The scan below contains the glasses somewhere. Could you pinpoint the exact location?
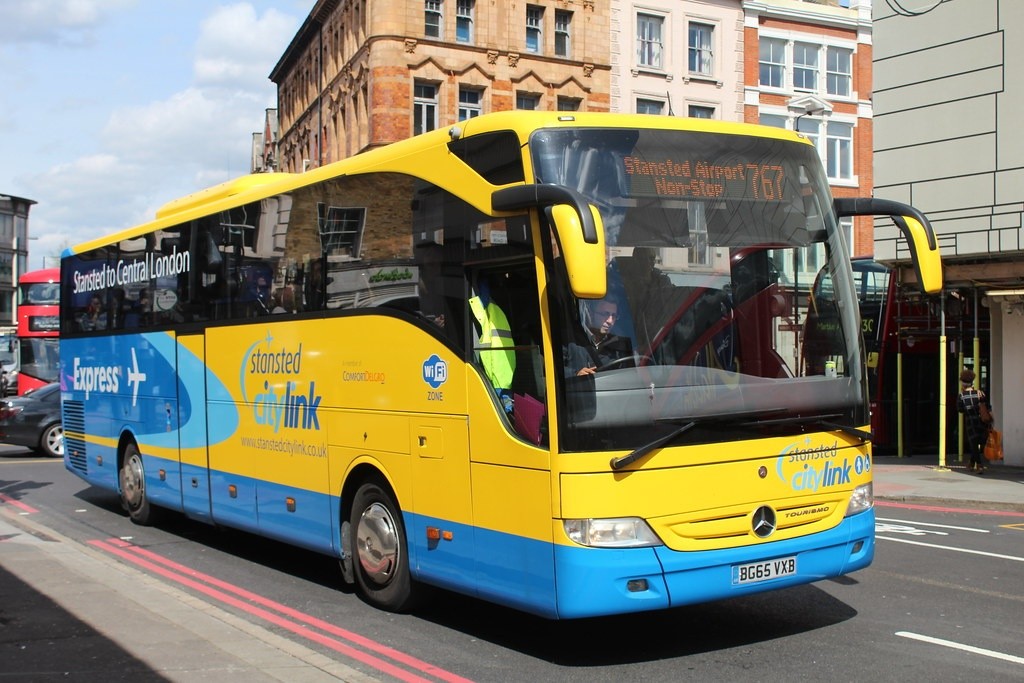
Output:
[593,311,621,321]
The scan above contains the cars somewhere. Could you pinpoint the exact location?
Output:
[0,382,64,458]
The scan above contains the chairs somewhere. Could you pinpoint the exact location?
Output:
[612,254,636,282]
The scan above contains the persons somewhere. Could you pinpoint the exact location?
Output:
[958,370,995,473]
[76,240,673,378]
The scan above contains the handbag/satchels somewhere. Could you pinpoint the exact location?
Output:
[984,427,1004,460]
[976,389,993,423]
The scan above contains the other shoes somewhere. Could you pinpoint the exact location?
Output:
[976,464,984,473]
[966,460,974,472]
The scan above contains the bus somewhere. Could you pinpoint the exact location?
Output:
[806,255,989,455]
[59,112,944,624]
[8,269,60,398]
